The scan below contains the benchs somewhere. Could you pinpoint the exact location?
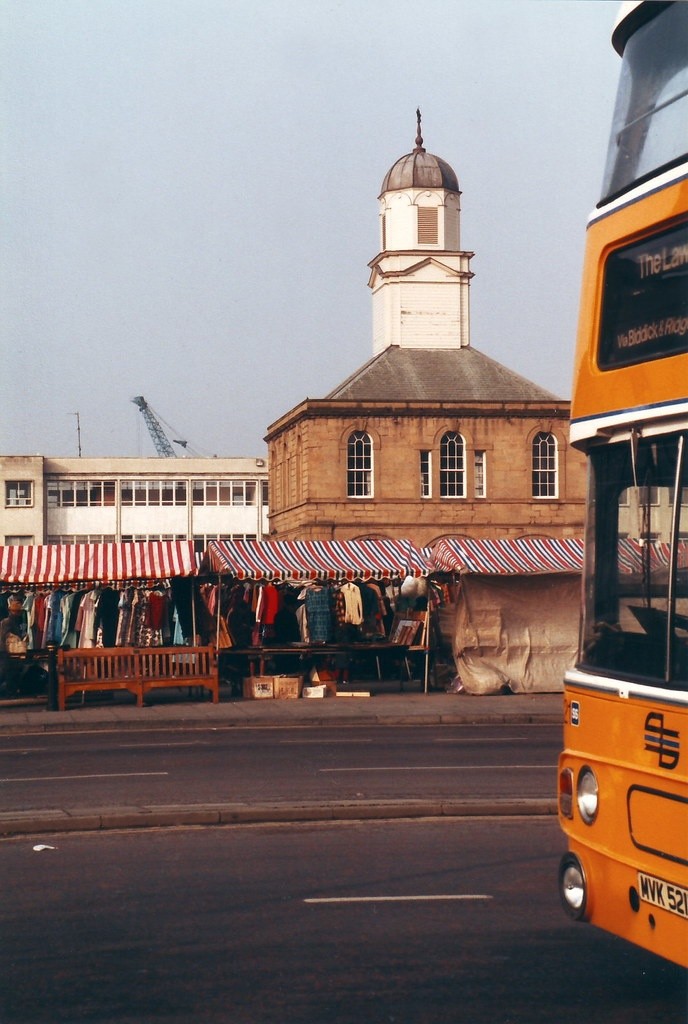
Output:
[58,644,219,710]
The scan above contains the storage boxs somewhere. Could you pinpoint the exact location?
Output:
[241,675,372,698]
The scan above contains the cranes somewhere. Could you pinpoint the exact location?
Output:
[132,393,204,454]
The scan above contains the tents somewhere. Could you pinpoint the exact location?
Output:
[432,538,688,696]
[0,540,197,666]
[203,538,431,694]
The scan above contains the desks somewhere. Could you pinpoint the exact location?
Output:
[222,643,424,691]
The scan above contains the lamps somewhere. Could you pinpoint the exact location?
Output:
[256,459,264,466]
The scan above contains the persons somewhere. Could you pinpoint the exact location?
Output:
[0,595,27,655]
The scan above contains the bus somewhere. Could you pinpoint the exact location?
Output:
[555,0,688,973]
